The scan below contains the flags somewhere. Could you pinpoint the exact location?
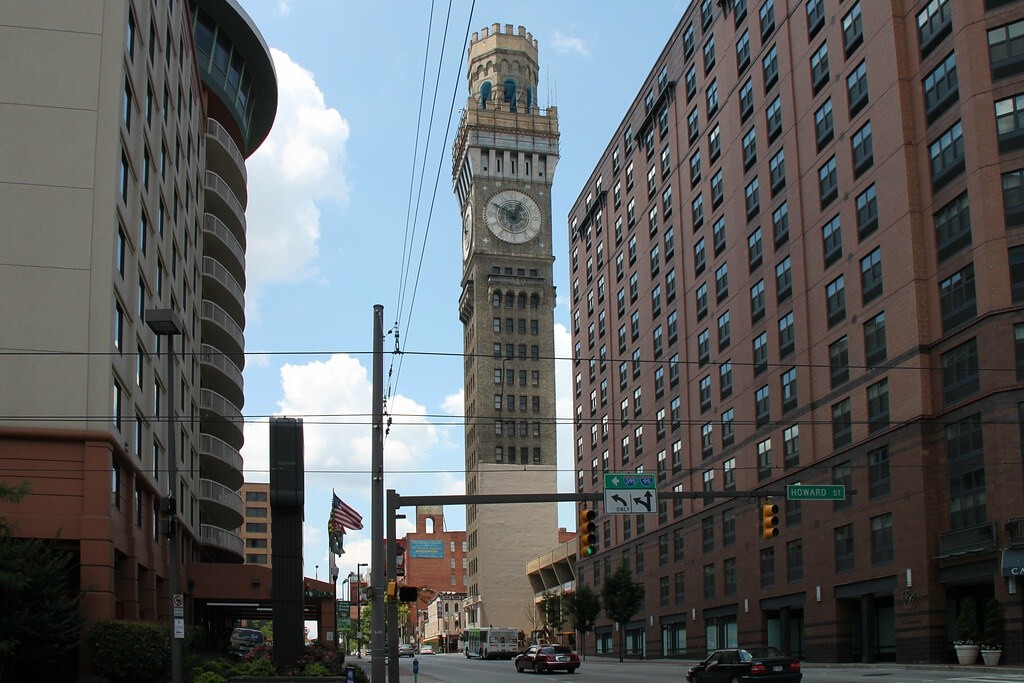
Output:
[333,492,364,530]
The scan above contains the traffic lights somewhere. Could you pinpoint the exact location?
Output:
[400,587,418,601]
[581,509,597,558]
[762,503,780,540]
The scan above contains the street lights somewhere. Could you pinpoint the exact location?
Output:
[358,563,367,658]
[142,309,183,683]
[422,588,446,654]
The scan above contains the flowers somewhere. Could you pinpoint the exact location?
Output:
[952,625,981,645]
[977,639,1002,650]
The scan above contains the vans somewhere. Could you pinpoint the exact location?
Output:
[229,629,266,658]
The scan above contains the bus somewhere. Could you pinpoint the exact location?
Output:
[461,624,518,661]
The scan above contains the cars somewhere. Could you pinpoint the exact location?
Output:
[421,645,433,654]
[515,644,581,673]
[686,647,803,683]
[399,643,415,658]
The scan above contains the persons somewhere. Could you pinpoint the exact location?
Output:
[518,629,526,646]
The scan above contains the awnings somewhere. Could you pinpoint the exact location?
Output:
[1001,548,1024,577]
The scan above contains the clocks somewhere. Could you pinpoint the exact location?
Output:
[462,200,473,263]
[482,188,546,246]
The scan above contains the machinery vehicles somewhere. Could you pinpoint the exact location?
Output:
[518,629,550,650]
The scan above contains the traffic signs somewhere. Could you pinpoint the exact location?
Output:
[787,484,846,499]
[604,474,659,514]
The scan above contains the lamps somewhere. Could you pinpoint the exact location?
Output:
[745,598,749,613]
[650,615,654,627]
[816,586,822,603]
[616,622,619,632]
[691,608,696,621]
[906,568,912,587]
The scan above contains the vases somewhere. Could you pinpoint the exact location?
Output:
[981,650,1003,667]
[954,644,981,665]
